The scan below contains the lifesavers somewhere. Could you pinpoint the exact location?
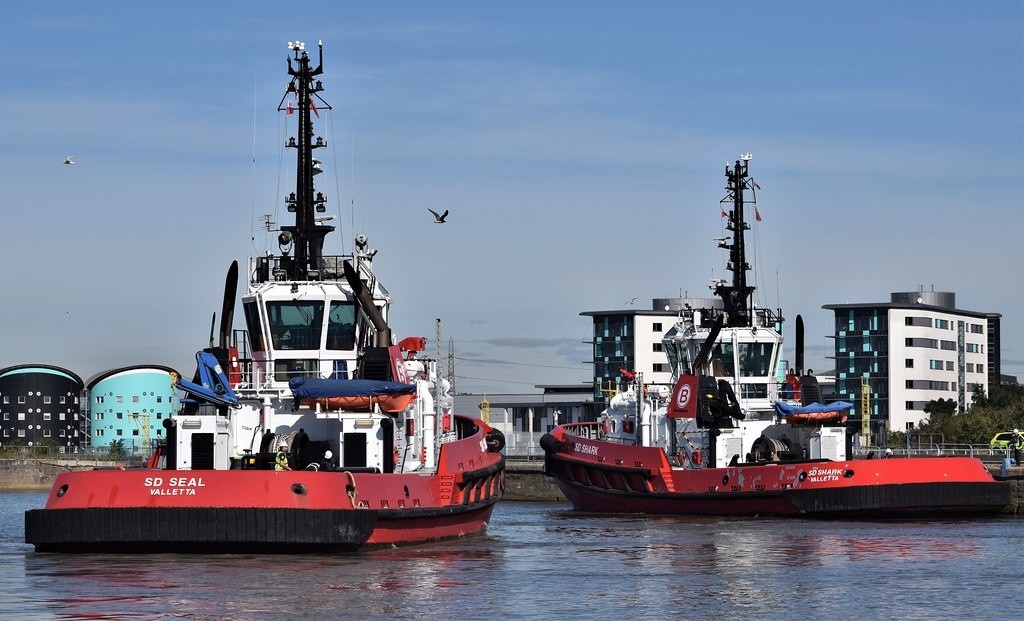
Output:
[600,420,608,434]
[675,451,684,464]
[421,362,427,380]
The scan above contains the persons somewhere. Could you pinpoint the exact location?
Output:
[1008,429,1024,467]
[275,441,292,470]
[867,451,874,459]
[321,451,335,472]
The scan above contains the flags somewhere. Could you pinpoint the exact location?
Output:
[756,211,762,221]
[753,181,761,190]
[288,101,293,114]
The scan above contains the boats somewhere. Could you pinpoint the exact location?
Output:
[781,407,851,427]
[300,385,419,416]
[26,36,509,560]
[540,154,1016,524]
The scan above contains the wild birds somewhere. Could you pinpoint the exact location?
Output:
[63,156,78,164]
[428,208,449,224]
[625,298,638,304]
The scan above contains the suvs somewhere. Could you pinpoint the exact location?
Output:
[988,430,1024,456]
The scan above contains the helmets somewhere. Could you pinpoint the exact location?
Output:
[279,441,288,447]
[1013,429,1018,433]
[886,448,891,453]
